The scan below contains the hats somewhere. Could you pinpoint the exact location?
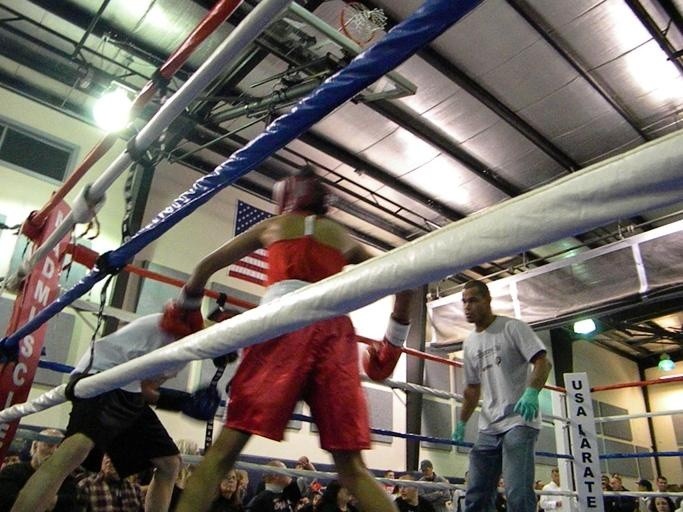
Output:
[420,461,431,470]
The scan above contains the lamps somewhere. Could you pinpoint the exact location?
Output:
[659,341,674,372]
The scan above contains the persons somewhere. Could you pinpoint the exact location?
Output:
[1,428,92,512]
[535,469,578,512]
[449,280,553,512]
[601,472,683,512]
[384,459,508,512]
[160,162,401,511]
[11,313,238,512]
[211,455,364,512]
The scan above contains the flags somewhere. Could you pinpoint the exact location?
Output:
[227,199,276,286]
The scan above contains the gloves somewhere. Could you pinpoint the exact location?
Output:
[514,386,541,421]
[159,285,205,338]
[155,386,217,417]
[363,315,413,380]
[452,421,464,442]
